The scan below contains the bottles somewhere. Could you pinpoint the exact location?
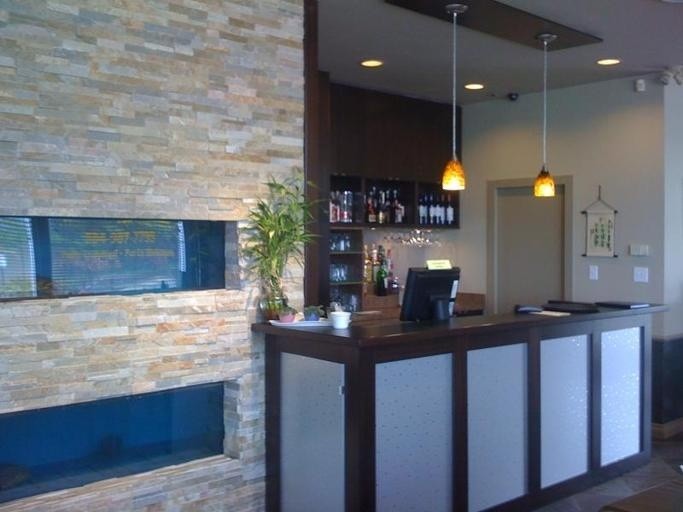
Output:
[439,195,444,225]
[330,264,349,282]
[330,234,351,251]
[370,250,381,283]
[418,194,424,227]
[329,191,353,223]
[378,191,390,225]
[392,188,404,225]
[446,195,455,226]
[434,195,441,225]
[364,245,372,283]
[429,196,435,225]
[376,259,388,296]
[424,193,429,225]
[367,197,377,225]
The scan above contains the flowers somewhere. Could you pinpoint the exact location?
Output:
[305,304,325,317]
[238,166,329,287]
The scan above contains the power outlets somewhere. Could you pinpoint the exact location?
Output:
[633,266,648,284]
[588,265,600,282]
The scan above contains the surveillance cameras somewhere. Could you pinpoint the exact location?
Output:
[508,92,518,100]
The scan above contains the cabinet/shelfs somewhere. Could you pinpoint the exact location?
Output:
[319,174,461,313]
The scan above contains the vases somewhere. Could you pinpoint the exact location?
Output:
[258,286,290,321]
[305,309,320,321]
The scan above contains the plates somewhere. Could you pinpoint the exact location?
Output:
[268,318,332,326]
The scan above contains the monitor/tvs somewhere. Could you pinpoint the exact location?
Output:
[399,267,460,323]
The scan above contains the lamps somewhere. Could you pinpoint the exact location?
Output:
[534,33,558,196]
[442,4,468,191]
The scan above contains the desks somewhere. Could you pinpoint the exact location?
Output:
[251,304,670,512]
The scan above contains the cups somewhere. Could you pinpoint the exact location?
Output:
[330,311,352,330]
[338,264,345,281]
[335,239,345,251]
[398,285,406,306]
[330,263,340,281]
